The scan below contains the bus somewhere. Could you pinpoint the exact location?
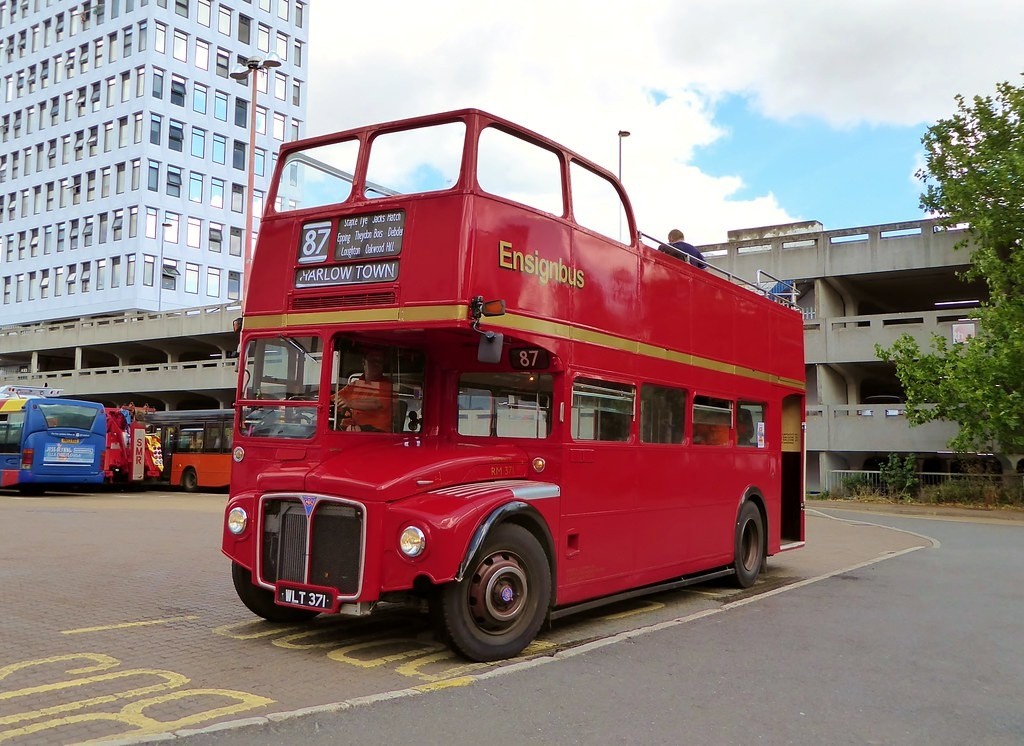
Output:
[0,385,107,497]
[142,410,312,493]
[219,107,809,665]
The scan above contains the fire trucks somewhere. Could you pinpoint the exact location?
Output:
[41,404,163,493]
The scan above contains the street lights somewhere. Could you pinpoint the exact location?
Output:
[228,50,281,429]
[158,222,173,319]
[617,131,631,184]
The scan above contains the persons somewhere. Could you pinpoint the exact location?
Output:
[330,351,396,433]
[658,229,709,271]
[693,402,754,446]
